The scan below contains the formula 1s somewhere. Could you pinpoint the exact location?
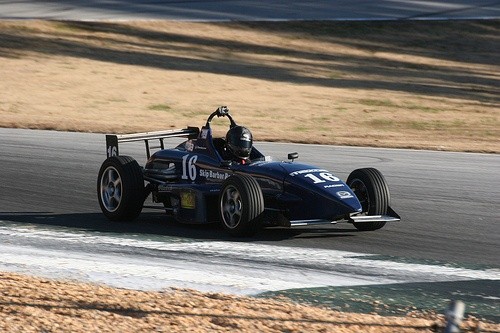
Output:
[96,105,403,237]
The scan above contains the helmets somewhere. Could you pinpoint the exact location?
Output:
[225,125,253,159]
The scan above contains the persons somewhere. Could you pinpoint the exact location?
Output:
[223,124,254,165]
